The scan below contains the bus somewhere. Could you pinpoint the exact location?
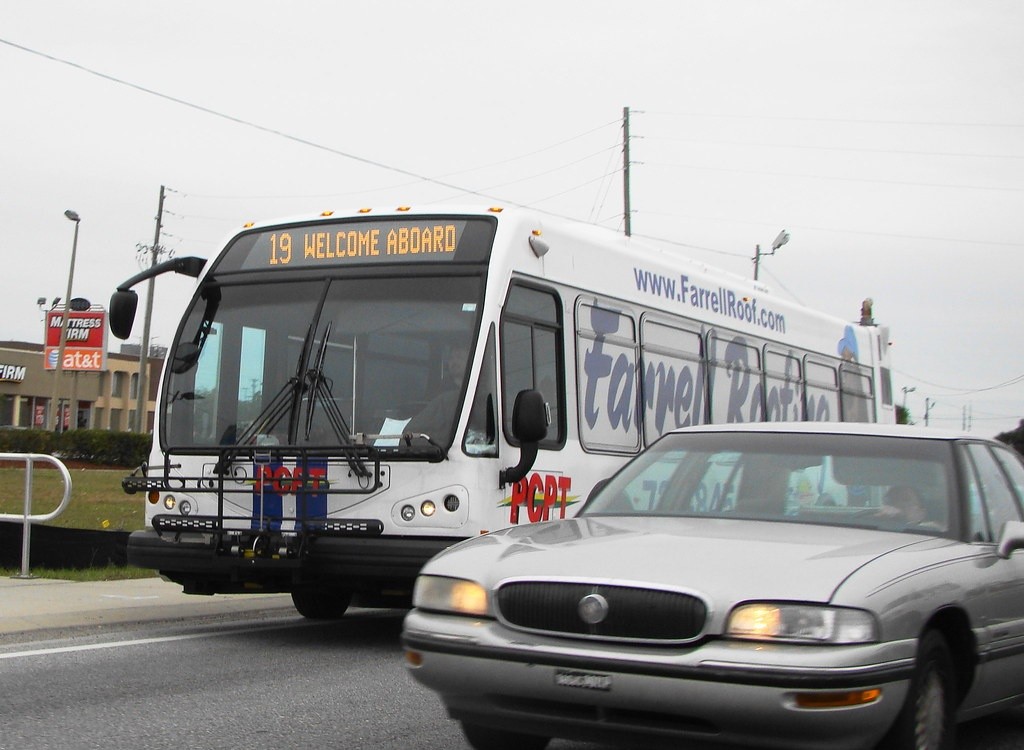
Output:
[110,207,891,630]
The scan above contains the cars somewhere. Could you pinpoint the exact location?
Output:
[401,421,1024,750]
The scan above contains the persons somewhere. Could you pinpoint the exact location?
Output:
[430,349,485,401]
[876,486,945,531]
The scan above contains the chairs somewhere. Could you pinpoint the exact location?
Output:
[738,467,789,517]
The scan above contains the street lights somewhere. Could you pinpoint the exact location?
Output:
[903,384,916,410]
[45,209,83,433]
[753,227,792,284]
[37,296,62,350]
